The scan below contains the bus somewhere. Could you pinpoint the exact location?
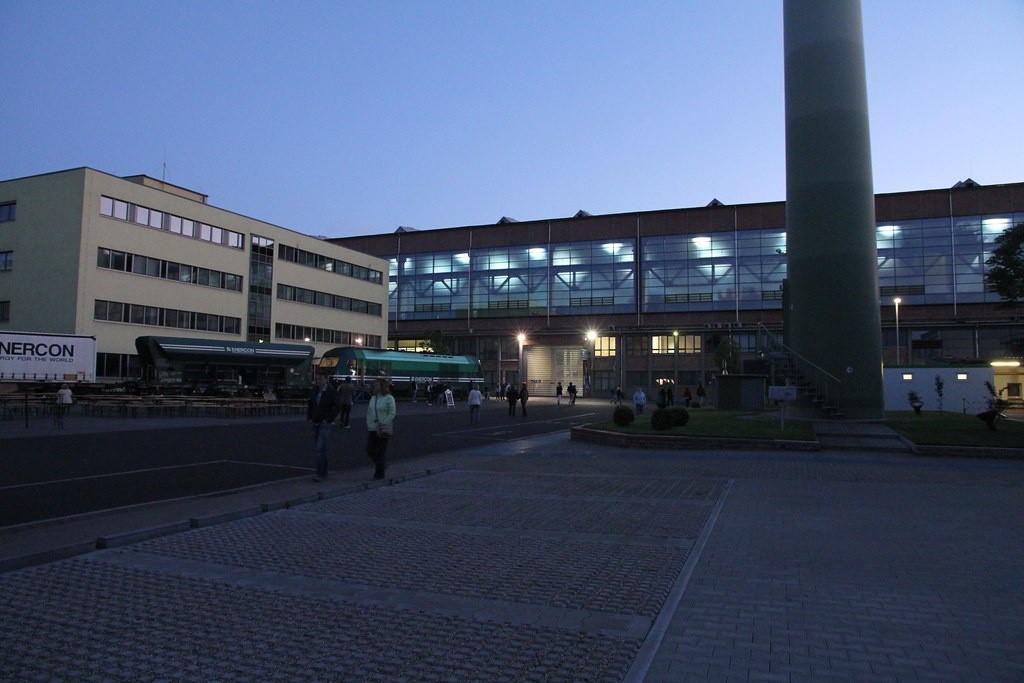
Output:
[131,335,485,400]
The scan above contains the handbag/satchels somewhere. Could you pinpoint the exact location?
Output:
[377,422,394,439]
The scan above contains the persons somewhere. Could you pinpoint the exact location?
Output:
[697,386,706,408]
[684,388,692,407]
[610,385,674,414]
[56,384,72,415]
[310,371,356,483]
[556,382,578,408]
[412,379,451,407]
[365,378,396,481]
[468,383,482,425]
[496,382,529,418]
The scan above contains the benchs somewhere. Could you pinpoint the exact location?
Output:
[0,394,308,420]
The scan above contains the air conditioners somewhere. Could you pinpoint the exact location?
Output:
[704,321,742,328]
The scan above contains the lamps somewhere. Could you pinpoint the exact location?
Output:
[776,249,783,254]
[911,401,923,414]
[977,410,998,431]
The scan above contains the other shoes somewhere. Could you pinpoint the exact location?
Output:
[343,425,352,429]
[312,475,321,481]
[371,473,385,481]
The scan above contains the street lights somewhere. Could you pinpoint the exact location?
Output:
[673,330,679,400]
[893,297,902,369]
